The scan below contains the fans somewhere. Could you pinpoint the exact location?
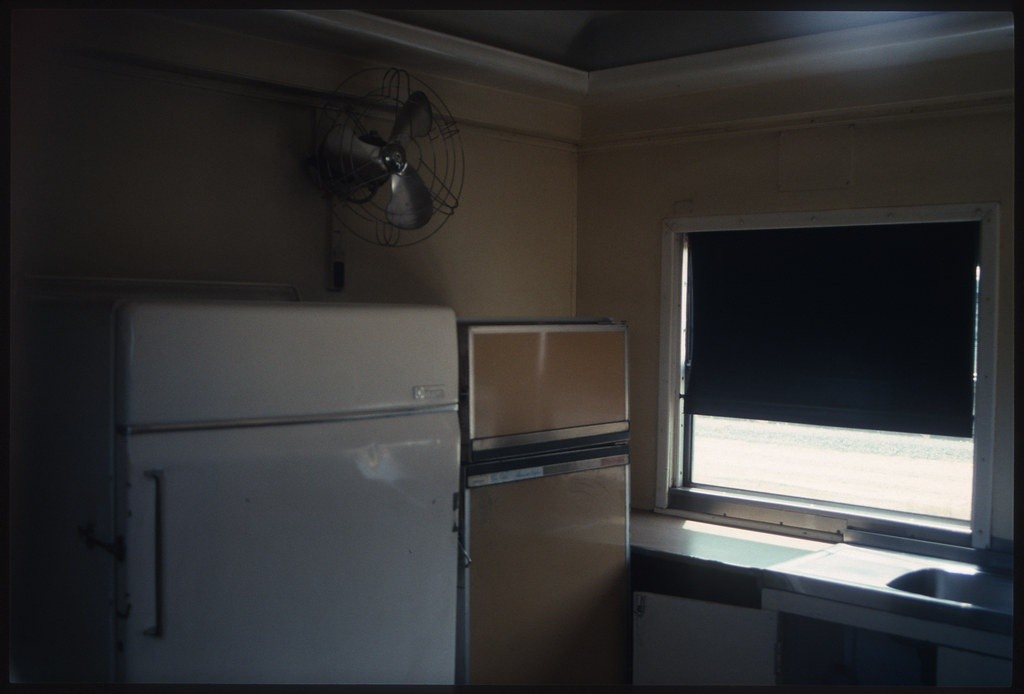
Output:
[311,64,466,290]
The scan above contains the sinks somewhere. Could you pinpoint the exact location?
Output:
[889,568,1013,611]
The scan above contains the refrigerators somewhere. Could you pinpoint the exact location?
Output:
[75,293,459,694]
